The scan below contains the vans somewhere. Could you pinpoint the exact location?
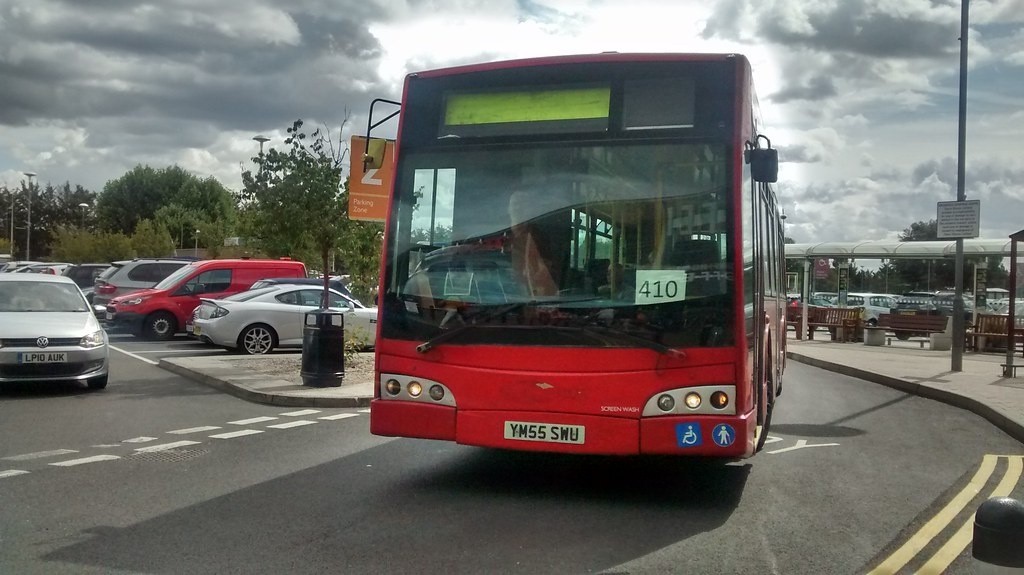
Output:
[105,256,307,342]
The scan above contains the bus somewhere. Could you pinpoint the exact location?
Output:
[343,49,798,476]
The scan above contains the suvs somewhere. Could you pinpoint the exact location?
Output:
[94,257,200,313]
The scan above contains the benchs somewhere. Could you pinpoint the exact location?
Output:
[807,307,861,342]
[964,314,1009,353]
[864,313,949,334]
[787,307,803,339]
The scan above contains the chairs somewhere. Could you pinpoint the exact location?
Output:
[586,239,721,301]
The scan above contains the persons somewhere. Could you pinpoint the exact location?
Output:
[598,262,635,303]
[498,191,561,296]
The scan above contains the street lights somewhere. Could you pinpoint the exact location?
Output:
[194,229,201,256]
[24,171,37,261]
[78,202,89,229]
[252,133,270,173]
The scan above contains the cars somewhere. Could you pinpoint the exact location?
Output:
[62,263,111,304]
[888,289,971,340]
[0,273,109,390]
[829,291,897,327]
[249,277,358,300]
[192,284,378,355]
[788,295,833,332]
[0,253,75,276]
[791,282,1024,329]
[308,268,379,296]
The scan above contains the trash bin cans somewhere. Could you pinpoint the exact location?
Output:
[299,307,345,387]
